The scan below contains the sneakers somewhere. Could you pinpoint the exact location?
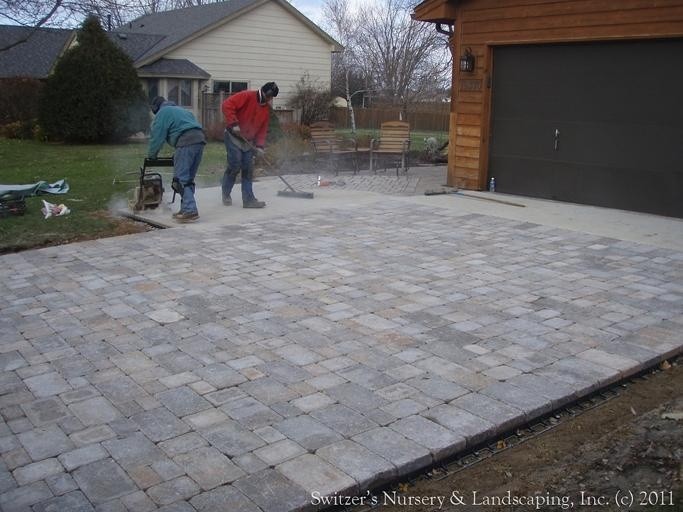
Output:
[171,209,200,225]
[221,191,234,206]
[242,198,267,209]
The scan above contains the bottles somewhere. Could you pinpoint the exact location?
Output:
[317,175,321,187]
[489,178,496,193]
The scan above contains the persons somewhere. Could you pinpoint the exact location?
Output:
[147,95,208,223]
[220,81,280,209]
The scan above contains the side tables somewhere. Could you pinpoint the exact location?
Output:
[342,146,370,176]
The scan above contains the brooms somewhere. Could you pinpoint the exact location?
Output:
[239,132,314,199]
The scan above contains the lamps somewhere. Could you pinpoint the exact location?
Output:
[458,47,474,72]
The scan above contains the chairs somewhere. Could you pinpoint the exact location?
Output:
[308,120,357,177]
[370,120,410,177]
